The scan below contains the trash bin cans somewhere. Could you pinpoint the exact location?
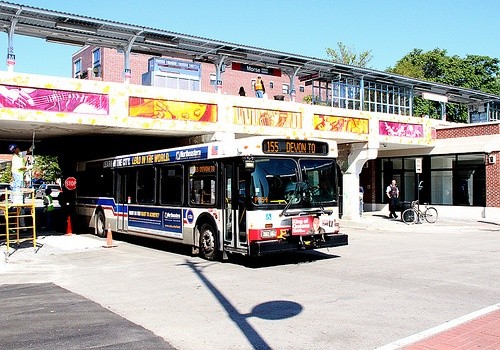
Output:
[399,202,415,222]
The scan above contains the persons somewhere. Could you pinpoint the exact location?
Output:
[9,144,35,208]
[254,75,266,98]
[386,180,400,219]
[44,188,54,236]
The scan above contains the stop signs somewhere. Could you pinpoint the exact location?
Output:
[65,177,77,190]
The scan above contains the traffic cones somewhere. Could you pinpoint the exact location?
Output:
[101,222,119,250]
[64,216,74,236]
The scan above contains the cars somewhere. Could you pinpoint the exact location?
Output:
[36,184,61,200]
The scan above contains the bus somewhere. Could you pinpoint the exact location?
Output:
[75,136,348,261]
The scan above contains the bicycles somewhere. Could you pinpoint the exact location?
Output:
[401,200,438,225]
